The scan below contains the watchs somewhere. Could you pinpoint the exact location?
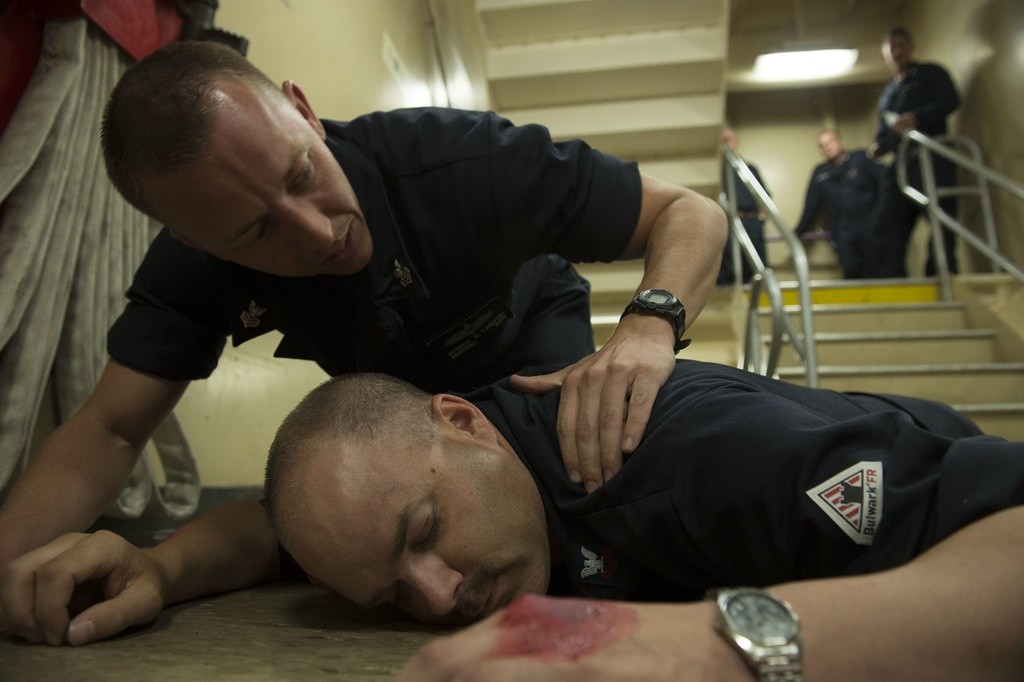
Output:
[614,287,692,357]
[701,584,805,680]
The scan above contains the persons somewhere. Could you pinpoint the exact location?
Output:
[793,130,892,280]
[0,39,731,574]
[865,24,963,278]
[719,125,771,284]
[0,358,1022,682]
